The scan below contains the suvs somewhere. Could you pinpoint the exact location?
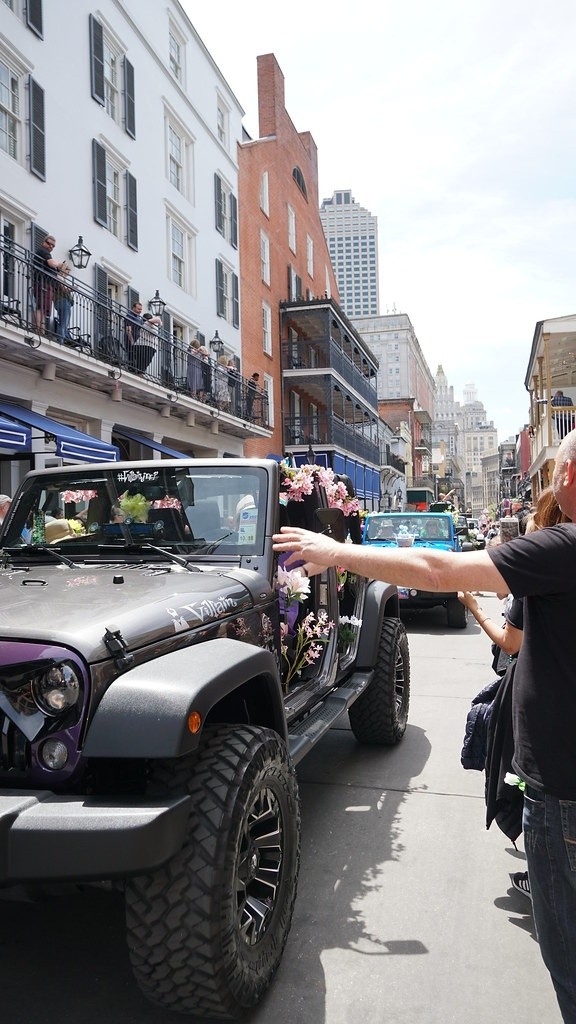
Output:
[363,512,469,629]
[1,454,411,1024]
[453,516,485,551]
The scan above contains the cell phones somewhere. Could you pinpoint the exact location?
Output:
[499,517,519,544]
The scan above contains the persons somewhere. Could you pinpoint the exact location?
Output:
[0,494,190,547]
[30,235,80,344]
[546,390,574,443]
[271,426,576,1024]
[246,373,259,414]
[124,302,162,379]
[187,339,238,410]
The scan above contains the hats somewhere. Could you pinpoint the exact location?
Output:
[379,519,394,528]
[44,519,97,545]
[0,495,13,506]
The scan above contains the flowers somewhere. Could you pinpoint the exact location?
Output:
[60,489,183,523]
[275,458,364,692]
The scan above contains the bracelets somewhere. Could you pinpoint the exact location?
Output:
[479,617,492,625]
[302,566,309,578]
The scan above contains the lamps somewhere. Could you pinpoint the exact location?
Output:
[210,420,219,434]
[187,413,195,428]
[210,330,224,352]
[43,362,57,381]
[69,236,91,269]
[112,388,122,401]
[148,290,166,317]
[161,405,171,418]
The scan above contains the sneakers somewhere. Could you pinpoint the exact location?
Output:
[512,871,531,898]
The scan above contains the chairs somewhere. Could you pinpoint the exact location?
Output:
[54,318,93,355]
[164,366,190,392]
[0,300,23,326]
[99,336,137,366]
[287,425,308,446]
[288,354,307,368]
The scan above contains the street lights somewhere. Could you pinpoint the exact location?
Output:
[520,484,526,508]
[396,487,403,512]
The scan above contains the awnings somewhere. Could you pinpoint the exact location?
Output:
[0,415,32,452]
[0,400,121,464]
[112,428,193,461]
